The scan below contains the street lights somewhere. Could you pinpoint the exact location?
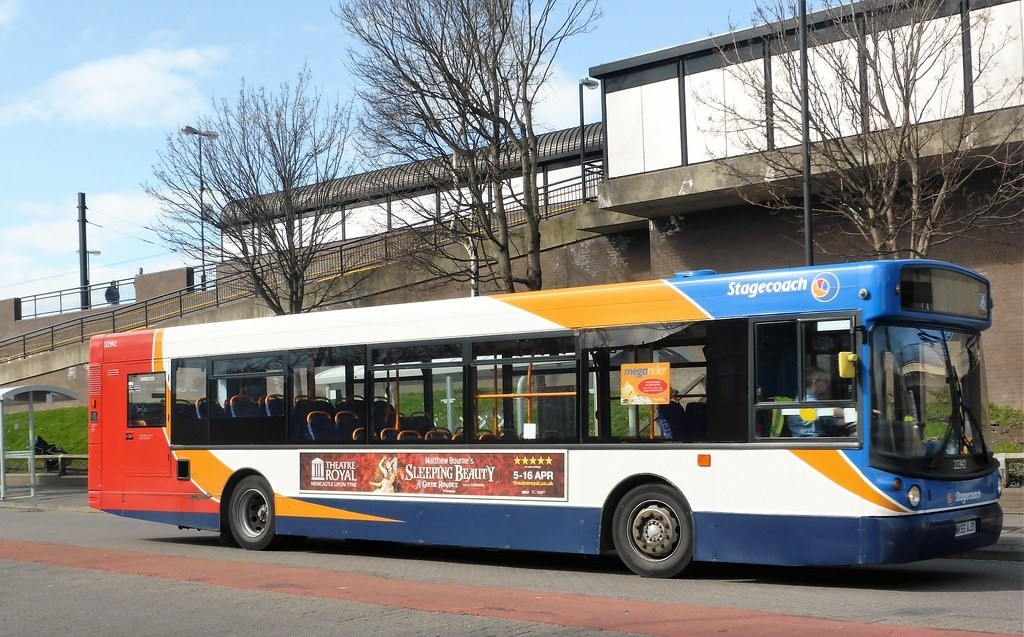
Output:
[86,249,101,284]
[576,77,601,204]
[179,123,220,290]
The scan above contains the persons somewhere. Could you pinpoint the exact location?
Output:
[105,280,119,305]
[787,367,846,438]
[133,268,143,287]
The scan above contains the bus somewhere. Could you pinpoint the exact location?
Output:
[85,258,1006,580]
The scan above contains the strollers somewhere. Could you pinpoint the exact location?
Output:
[35,434,73,473]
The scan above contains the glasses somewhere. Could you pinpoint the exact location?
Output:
[817,379,830,384]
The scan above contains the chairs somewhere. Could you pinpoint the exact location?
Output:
[658,401,706,443]
[162,393,560,444]
